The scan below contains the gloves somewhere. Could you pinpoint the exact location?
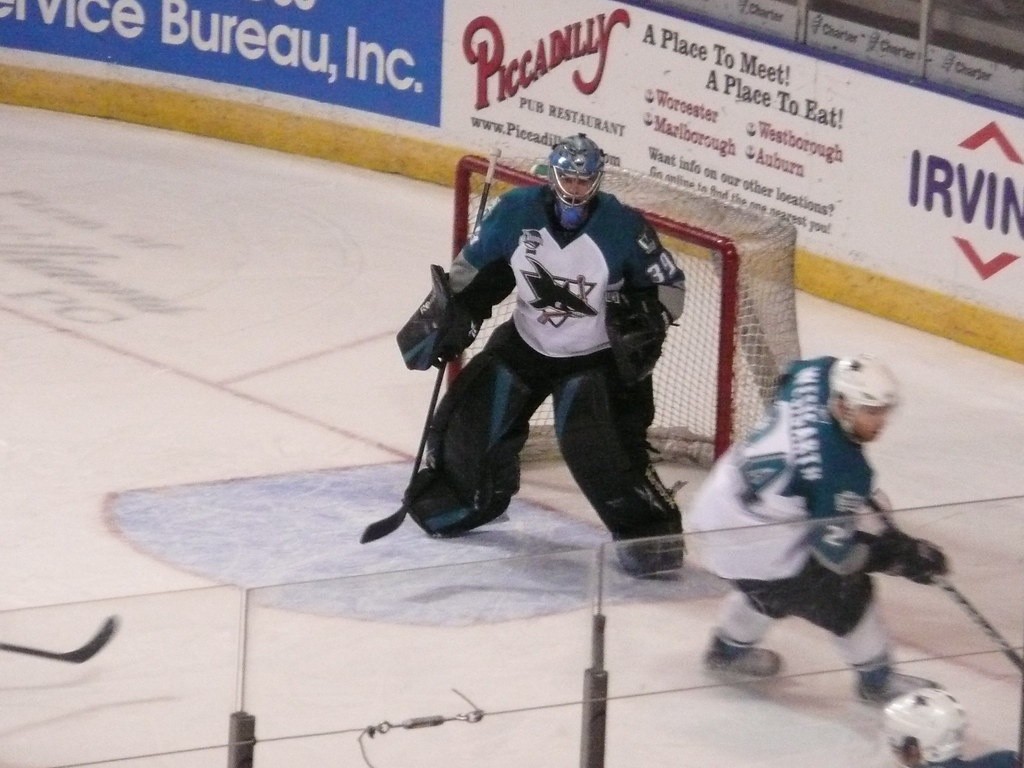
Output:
[863,526,947,585]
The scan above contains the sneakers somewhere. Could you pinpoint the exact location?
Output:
[702,634,780,681]
[853,652,943,709]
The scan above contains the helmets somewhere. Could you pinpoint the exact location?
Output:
[548,133,609,230]
[829,352,902,406]
[883,688,965,762]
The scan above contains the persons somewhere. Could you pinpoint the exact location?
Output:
[683,354,950,717]
[881,689,1024,768]
[395,134,687,577]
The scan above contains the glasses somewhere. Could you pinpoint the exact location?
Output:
[560,174,594,185]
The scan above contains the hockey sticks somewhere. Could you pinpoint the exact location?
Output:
[866,498,1023,674]
[358,145,502,545]
[0,613,120,666]
